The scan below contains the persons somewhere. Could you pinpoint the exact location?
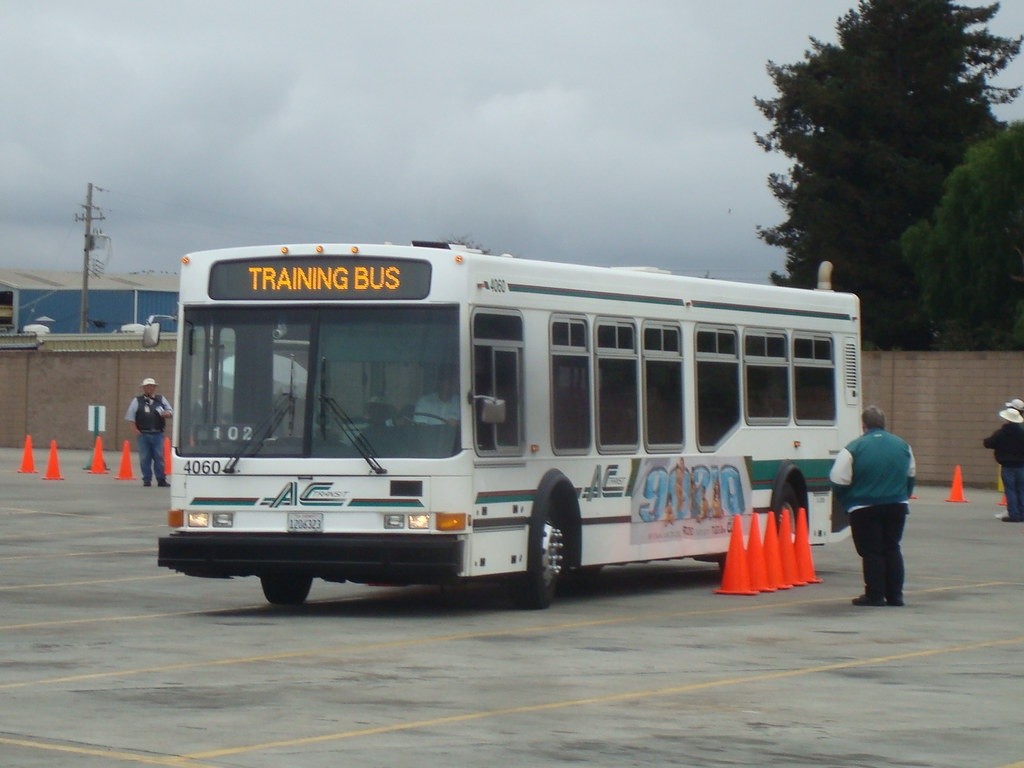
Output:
[983,398,1024,522]
[123,378,173,487]
[829,406,916,607]
[397,364,461,427]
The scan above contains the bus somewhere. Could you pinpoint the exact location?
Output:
[141,242,863,610]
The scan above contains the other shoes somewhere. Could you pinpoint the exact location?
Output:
[1002,515,1024,522]
[887,595,904,606]
[996,511,1009,519]
[143,479,151,486]
[158,480,170,486]
[852,596,885,606]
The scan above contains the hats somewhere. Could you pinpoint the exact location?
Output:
[141,378,158,386]
[999,408,1024,423]
[1004,399,1024,410]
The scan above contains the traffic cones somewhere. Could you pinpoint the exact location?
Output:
[945,466,967,502]
[794,506,822,584]
[998,494,1007,505]
[40,439,64,479]
[716,514,758,596]
[87,437,109,474]
[747,515,773,593]
[765,515,788,590]
[18,435,38,473]
[163,436,171,476]
[115,440,137,479]
[781,511,804,587]
